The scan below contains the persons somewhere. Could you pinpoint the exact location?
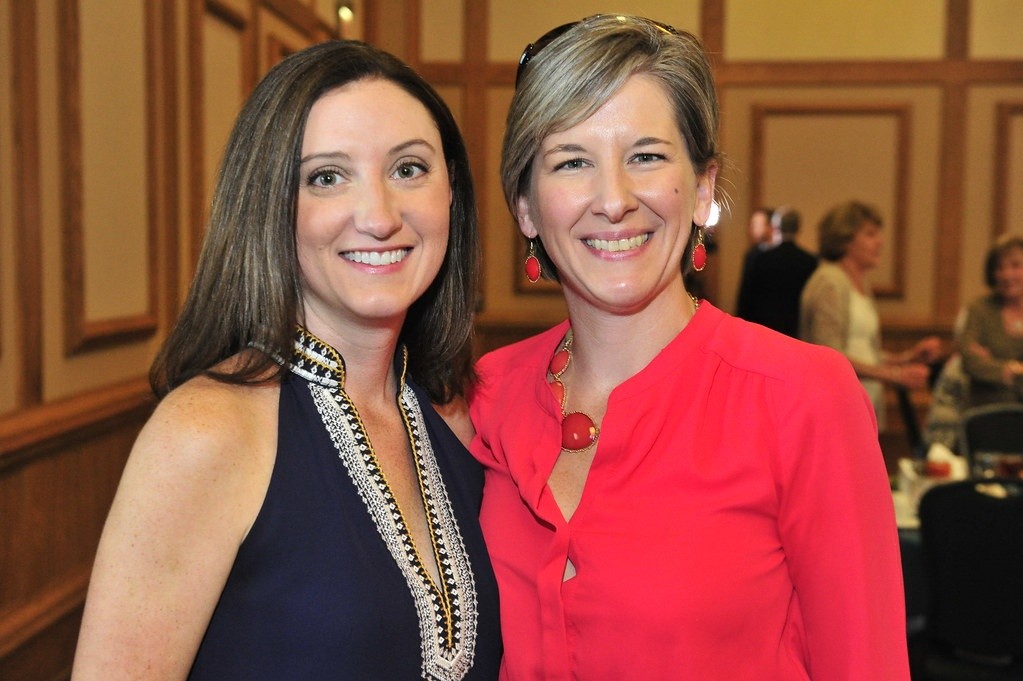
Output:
[957,231,1023,405]
[464,12,942,681]
[69,39,504,680]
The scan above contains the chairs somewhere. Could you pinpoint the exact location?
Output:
[894,376,1023,461]
[917,475,1022,681]
